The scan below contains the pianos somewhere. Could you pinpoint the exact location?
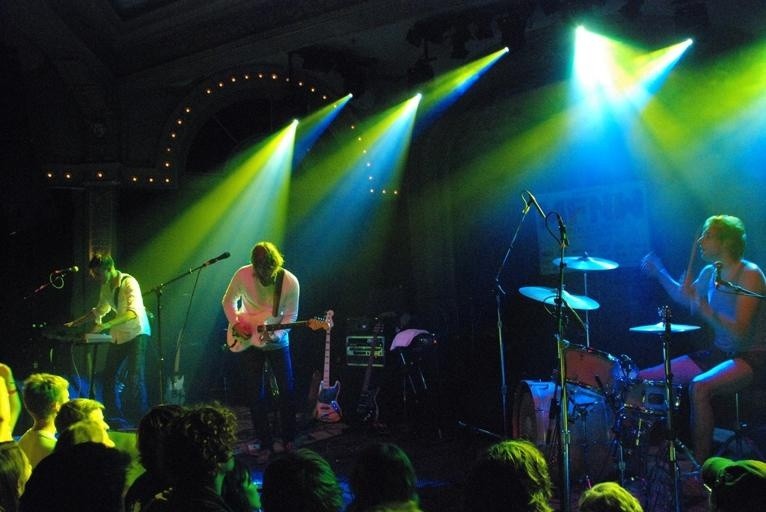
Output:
[42,333,113,345]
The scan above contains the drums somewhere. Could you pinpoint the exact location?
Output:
[512,379,624,511]
[548,339,622,394]
[622,380,688,416]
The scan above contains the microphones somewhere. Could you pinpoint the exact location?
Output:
[524,189,546,219]
[204,252,230,265]
[566,304,584,328]
[556,214,568,248]
[713,262,722,289]
[54,266,79,275]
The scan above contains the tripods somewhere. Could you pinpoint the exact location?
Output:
[647,336,702,512]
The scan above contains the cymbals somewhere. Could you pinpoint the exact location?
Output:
[553,256,619,271]
[629,322,701,334]
[518,285,600,310]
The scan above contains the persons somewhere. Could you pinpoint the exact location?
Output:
[222,241,300,440]
[257,450,342,510]
[478,438,557,511]
[637,215,765,471]
[349,443,421,511]
[64,252,151,428]
[2,361,261,511]
[577,482,644,511]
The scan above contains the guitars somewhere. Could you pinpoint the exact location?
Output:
[305,309,343,425]
[227,315,330,352]
[163,342,185,406]
[351,335,381,425]
